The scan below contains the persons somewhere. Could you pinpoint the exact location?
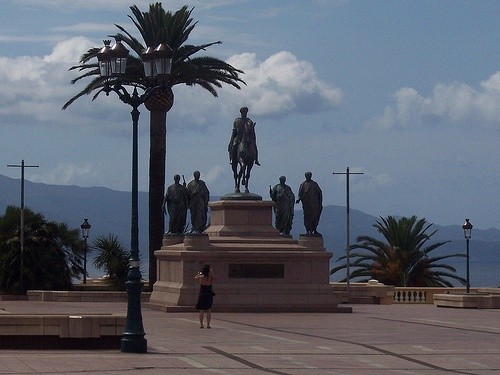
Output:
[161,174,187,233]
[227,106,260,166]
[195,264,216,329]
[187,170,210,234]
[269,176,295,238]
[296,171,322,237]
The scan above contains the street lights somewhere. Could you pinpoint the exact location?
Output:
[462,218,473,294]
[96,35,173,354]
[80,218,92,284]
